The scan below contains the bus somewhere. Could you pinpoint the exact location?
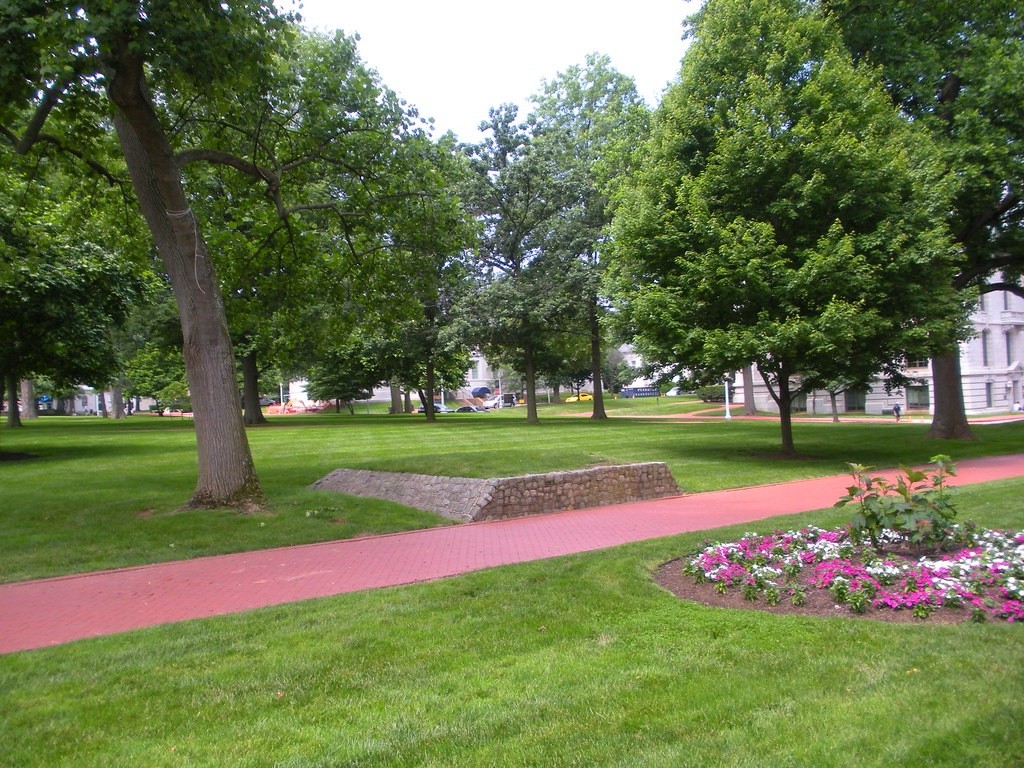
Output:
[619,387,660,397]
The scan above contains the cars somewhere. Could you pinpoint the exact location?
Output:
[418,404,455,413]
[484,395,517,409]
[259,393,276,406]
[565,393,594,401]
[456,405,490,413]
[666,387,692,396]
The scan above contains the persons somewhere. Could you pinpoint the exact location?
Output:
[893,402,902,423]
[1013,402,1024,411]
